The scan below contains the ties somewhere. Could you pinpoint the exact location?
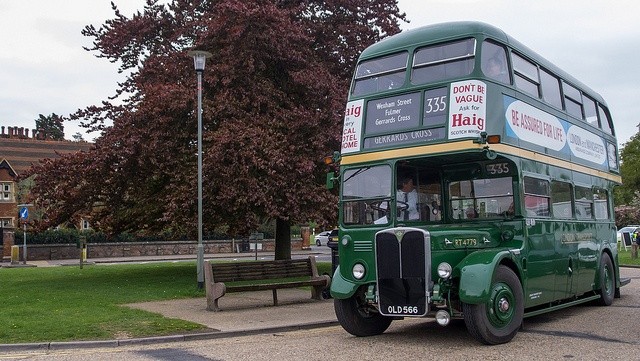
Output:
[404,191,409,221]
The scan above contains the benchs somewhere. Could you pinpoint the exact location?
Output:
[203,256,332,309]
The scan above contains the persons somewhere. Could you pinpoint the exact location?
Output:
[487,56,507,81]
[376,177,421,222]
[636,233,640,249]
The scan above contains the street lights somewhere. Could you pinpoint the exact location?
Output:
[186,47,215,288]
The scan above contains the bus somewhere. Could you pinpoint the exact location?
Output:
[324,18,633,346]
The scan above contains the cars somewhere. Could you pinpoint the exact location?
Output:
[617,225,640,242]
[314,231,332,247]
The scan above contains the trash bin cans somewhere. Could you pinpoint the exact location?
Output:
[322,229,338,279]
[242,238,250,252]
